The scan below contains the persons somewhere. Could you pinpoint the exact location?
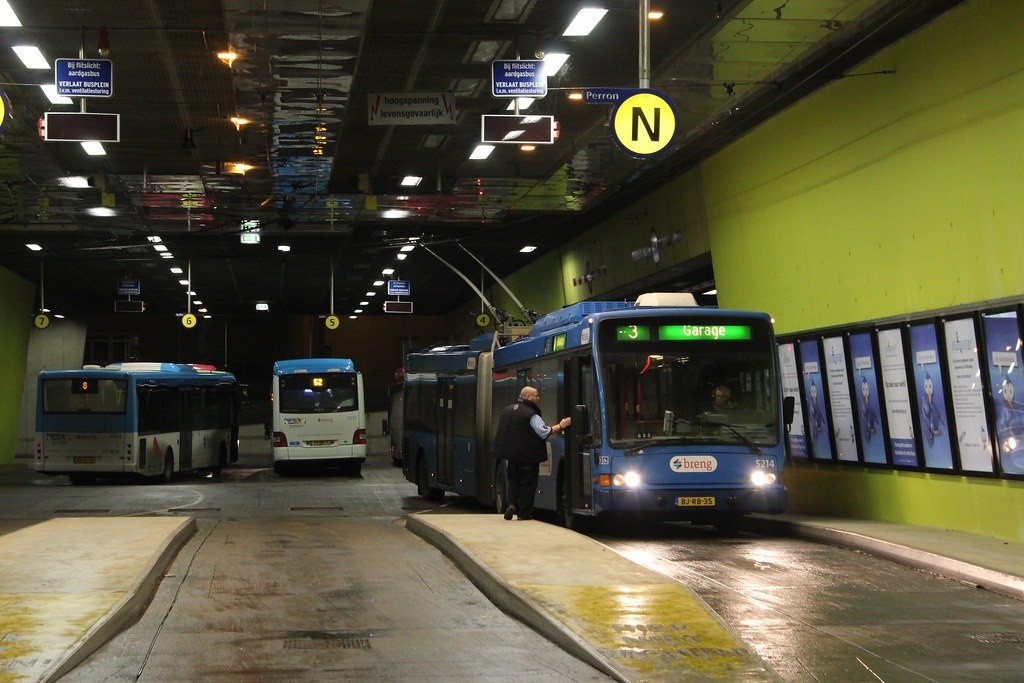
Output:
[492,386,572,521]
[711,384,735,413]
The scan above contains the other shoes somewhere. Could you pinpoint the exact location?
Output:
[517,513,532,519]
[504,504,515,520]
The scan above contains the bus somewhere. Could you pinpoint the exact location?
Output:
[33,361,249,485]
[381,368,404,466]
[402,293,795,539]
[270,358,367,476]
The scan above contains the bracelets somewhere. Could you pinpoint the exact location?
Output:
[559,423,563,430]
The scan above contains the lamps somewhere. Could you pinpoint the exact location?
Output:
[468,140,496,160]
[560,0,611,37]
[0,0,25,28]
[9,32,53,72]
[39,76,75,105]
[545,47,571,77]
[81,141,109,156]
[505,97,537,112]
